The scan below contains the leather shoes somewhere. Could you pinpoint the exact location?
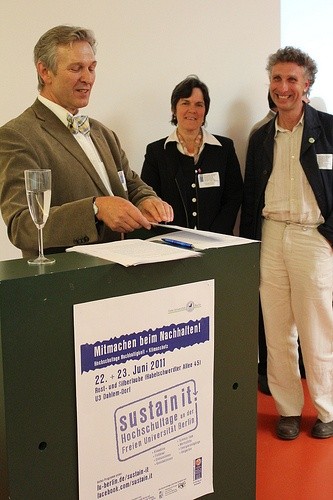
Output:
[311,419,333,439]
[276,416,301,439]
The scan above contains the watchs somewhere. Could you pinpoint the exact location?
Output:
[93,198,98,215]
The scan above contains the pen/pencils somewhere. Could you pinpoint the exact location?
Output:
[161,238,194,249]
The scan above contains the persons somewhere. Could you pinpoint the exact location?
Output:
[0,23,174,260]
[138,73,243,239]
[239,45,333,440]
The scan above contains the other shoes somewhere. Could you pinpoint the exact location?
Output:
[260,377,272,396]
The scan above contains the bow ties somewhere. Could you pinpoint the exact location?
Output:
[66,113,90,136]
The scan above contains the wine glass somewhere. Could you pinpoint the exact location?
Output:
[24,169,56,264]
